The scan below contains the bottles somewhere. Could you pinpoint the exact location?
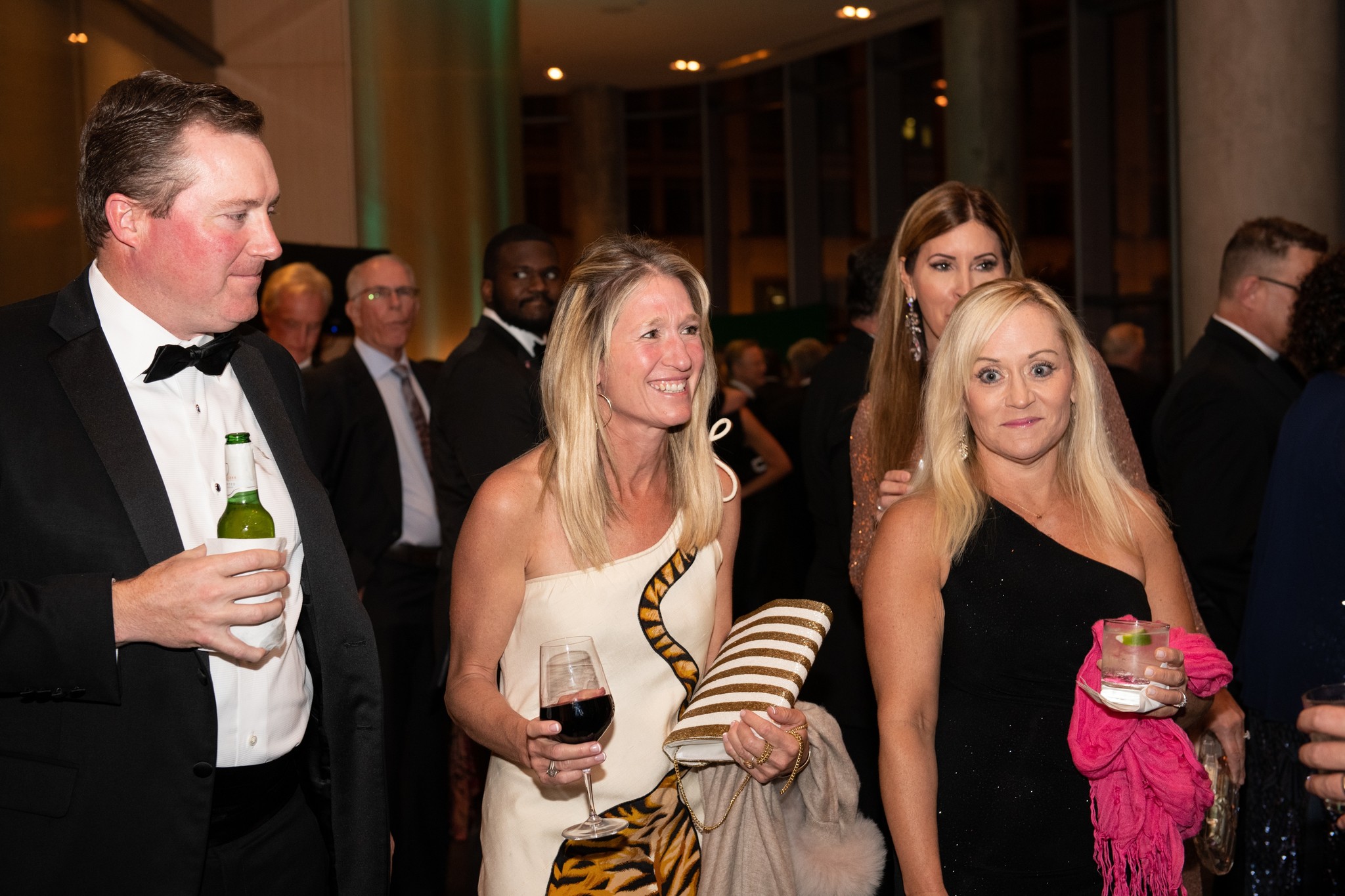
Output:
[220,430,285,654]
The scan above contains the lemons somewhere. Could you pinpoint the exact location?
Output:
[1115,626,1153,646]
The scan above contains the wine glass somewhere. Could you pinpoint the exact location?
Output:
[536,635,630,842]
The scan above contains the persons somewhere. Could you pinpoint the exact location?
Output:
[707,216,1345,895]
[426,226,564,550]
[849,185,1152,603]
[444,233,810,896]
[301,253,449,896]
[0,67,389,896]
[261,261,333,372]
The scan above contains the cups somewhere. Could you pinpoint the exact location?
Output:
[1099,613,1168,712]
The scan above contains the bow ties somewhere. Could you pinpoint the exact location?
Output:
[142,330,241,385]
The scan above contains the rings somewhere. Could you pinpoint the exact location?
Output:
[1342,773,1345,793]
[1171,689,1188,708]
[744,755,755,769]
[546,760,561,777]
[875,498,885,512]
[986,485,1063,519]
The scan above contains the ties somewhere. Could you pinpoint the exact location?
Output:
[391,365,433,476]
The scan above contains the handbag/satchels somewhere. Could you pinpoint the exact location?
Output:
[1189,730,1238,876]
[662,600,834,832]
